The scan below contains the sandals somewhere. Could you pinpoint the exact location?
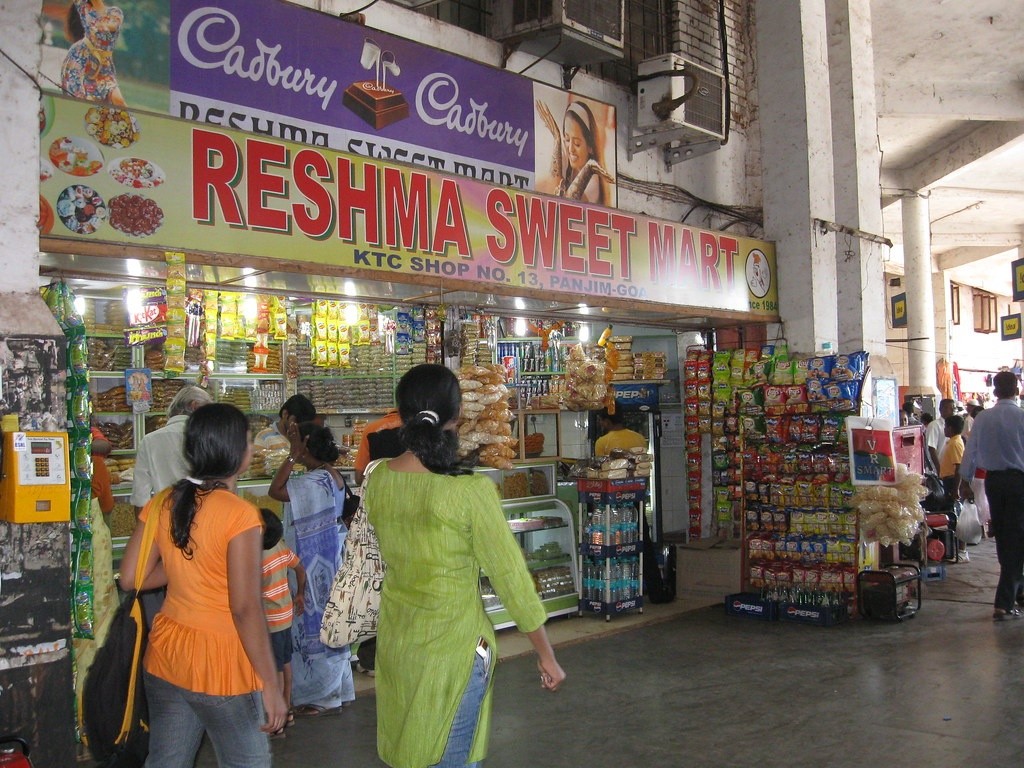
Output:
[993,609,1024,620]
[268,710,295,739]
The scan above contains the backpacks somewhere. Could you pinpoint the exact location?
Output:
[82,486,174,767]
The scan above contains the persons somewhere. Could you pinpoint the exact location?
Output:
[134,388,402,735]
[959,373,1024,622]
[89,427,115,533]
[900,399,994,565]
[538,102,612,205]
[369,363,565,768]
[117,408,288,768]
[594,401,666,604]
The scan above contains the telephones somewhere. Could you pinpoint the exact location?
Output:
[5,431,72,521]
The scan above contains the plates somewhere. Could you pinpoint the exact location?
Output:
[40,195,55,236]
[40,156,54,182]
[83,107,142,149]
[39,94,57,138]
[56,184,106,235]
[106,157,165,188]
[106,193,164,238]
[48,136,105,177]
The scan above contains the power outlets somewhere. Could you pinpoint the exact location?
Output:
[527,414,543,423]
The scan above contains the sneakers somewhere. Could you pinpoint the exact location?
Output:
[948,551,970,562]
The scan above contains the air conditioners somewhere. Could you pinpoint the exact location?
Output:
[638,53,725,144]
[491,0,625,49]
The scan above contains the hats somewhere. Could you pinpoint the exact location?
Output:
[968,399,980,406]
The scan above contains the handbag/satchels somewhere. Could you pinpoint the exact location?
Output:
[955,499,982,544]
[318,458,387,648]
[342,495,360,527]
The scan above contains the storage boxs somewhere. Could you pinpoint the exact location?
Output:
[726,592,787,620]
[779,601,848,626]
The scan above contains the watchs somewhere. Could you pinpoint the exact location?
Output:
[286,455,295,465]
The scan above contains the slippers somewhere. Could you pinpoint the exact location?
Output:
[294,704,343,717]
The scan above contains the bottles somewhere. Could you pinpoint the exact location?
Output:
[584,500,639,605]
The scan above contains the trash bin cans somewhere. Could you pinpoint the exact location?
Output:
[645,542,679,603]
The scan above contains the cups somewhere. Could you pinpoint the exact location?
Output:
[381,49,401,77]
[360,37,381,70]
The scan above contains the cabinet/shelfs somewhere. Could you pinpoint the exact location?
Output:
[111,461,579,662]
[73,289,582,494]
[738,366,879,621]
[577,477,647,622]
